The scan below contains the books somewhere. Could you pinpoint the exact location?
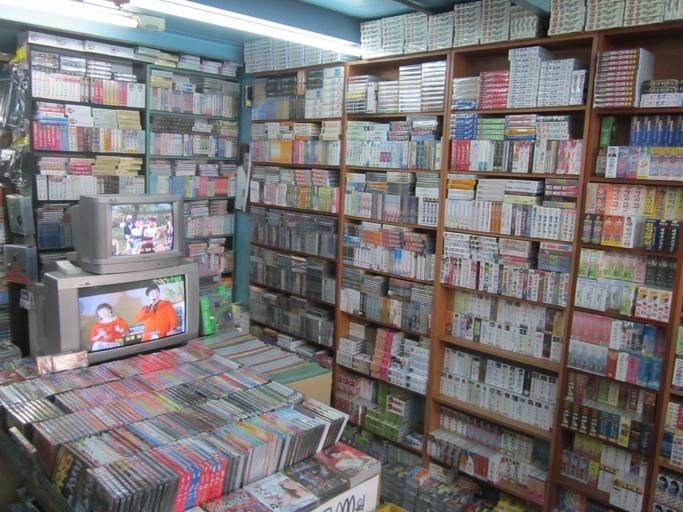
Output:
[427,48,587,498]
[322,0,683,64]
[2,32,346,369]
[556,49,683,511]
[2,326,537,511]
[335,62,448,451]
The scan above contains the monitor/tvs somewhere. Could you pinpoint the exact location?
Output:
[34,260,199,365]
[72,193,183,275]
